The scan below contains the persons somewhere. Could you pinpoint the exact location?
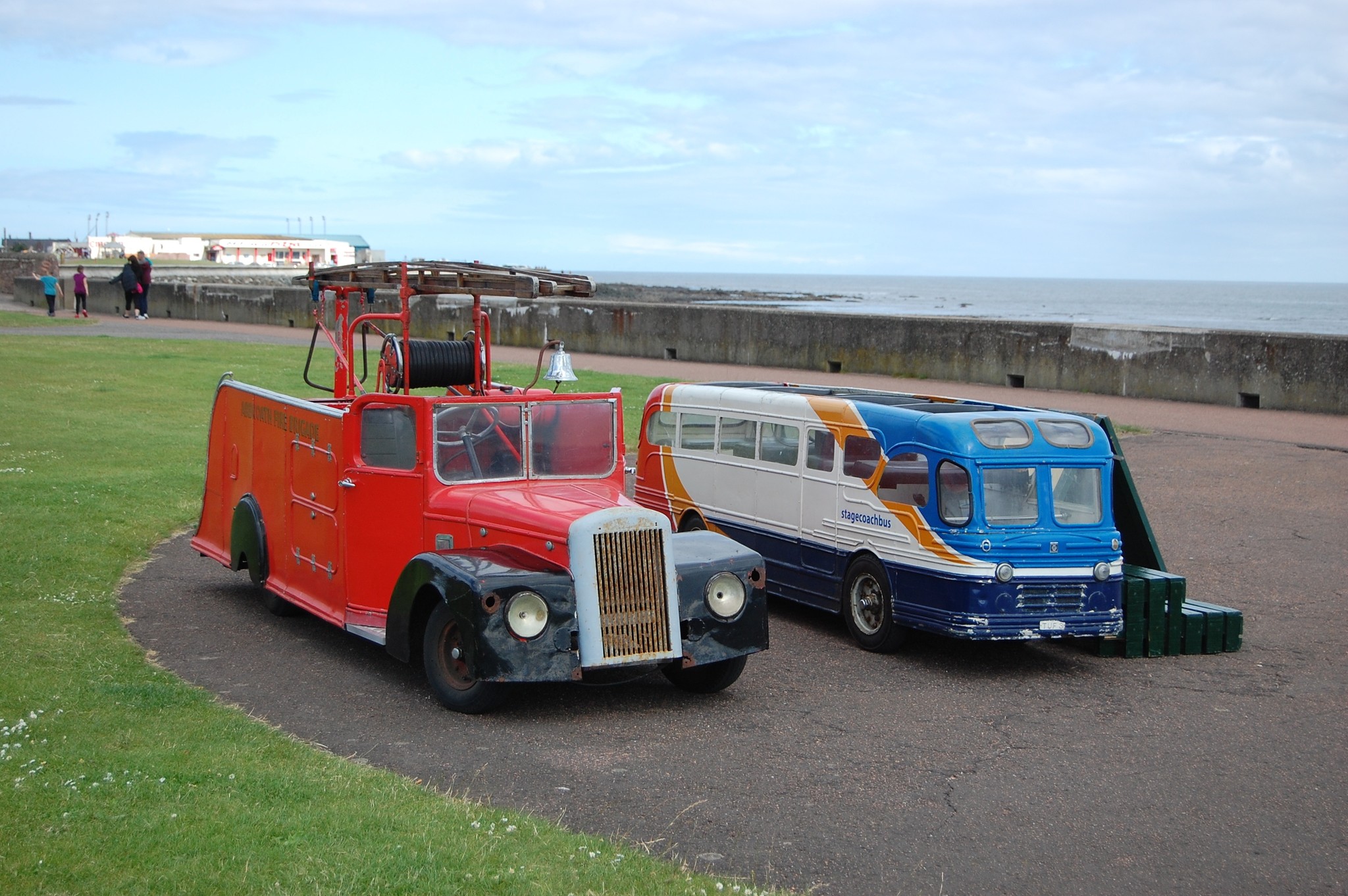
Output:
[137,251,152,319]
[73,265,89,318]
[110,255,146,320]
[31,268,63,316]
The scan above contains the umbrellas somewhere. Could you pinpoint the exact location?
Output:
[210,244,224,251]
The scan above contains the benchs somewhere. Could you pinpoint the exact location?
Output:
[852,459,1029,485]
[655,437,881,476]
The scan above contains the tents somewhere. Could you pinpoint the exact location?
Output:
[105,240,124,250]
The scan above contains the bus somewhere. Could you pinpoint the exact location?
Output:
[632,381,1124,657]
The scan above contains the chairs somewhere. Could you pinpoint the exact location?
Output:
[358,408,418,472]
[896,484,945,517]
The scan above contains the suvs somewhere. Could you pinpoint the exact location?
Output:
[190,259,771,716]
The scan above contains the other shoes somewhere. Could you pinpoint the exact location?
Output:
[73,314,79,318]
[138,315,146,320]
[121,314,129,319]
[47,311,55,316]
[144,313,149,319]
[135,316,139,320]
[82,309,88,317]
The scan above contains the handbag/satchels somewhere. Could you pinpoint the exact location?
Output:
[131,283,143,293]
